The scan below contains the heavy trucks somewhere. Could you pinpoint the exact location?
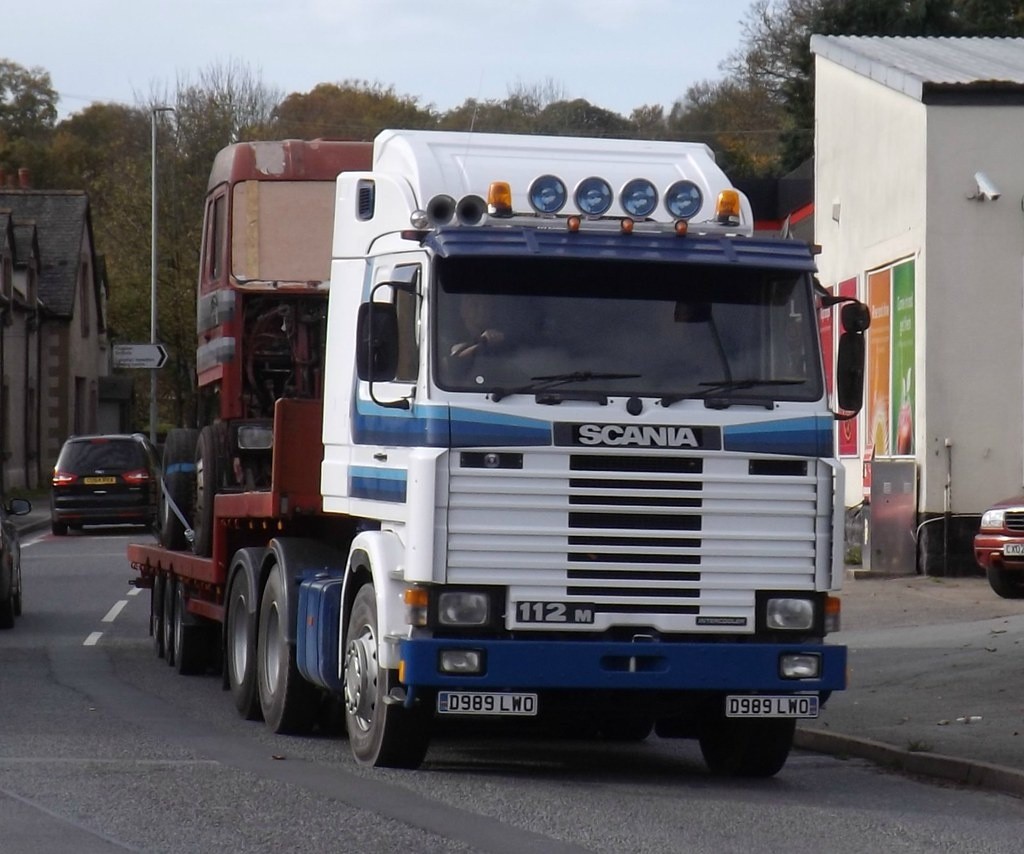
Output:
[126,127,871,779]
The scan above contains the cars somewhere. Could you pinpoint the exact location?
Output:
[975,495,1024,596]
[0,494,33,629]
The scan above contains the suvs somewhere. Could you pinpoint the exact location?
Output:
[49,432,158,535]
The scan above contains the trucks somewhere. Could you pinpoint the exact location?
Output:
[155,140,375,551]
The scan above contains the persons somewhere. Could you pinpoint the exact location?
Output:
[447,293,505,360]
